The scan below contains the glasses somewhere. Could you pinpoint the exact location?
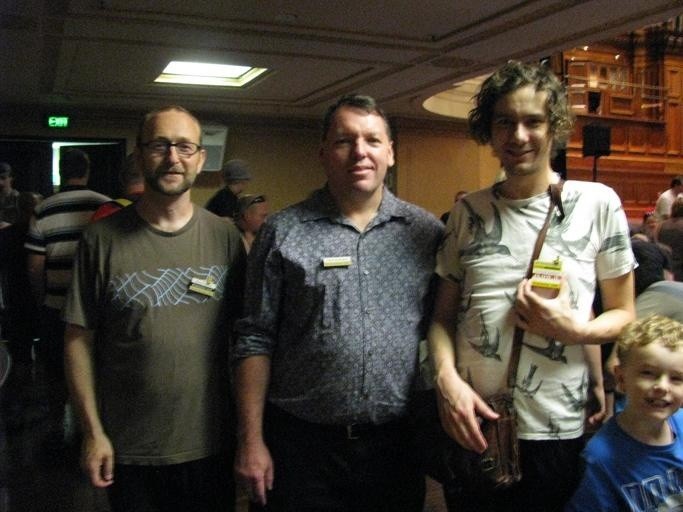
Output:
[138,138,204,157]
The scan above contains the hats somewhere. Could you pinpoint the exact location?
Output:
[222,159,251,184]
[234,191,268,222]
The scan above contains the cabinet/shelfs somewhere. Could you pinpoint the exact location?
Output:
[566,153,682,219]
[566,50,683,151]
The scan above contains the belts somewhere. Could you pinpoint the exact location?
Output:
[264,408,418,446]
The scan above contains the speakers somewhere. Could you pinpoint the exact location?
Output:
[582,120,612,157]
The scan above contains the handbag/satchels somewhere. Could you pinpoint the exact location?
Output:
[429,392,524,489]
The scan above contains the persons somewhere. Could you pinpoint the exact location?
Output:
[581,176,683,425]
[0,191,52,405]
[0,161,19,227]
[91,152,146,220]
[229,92,445,512]
[440,190,470,226]
[570,314,683,511]
[428,58,640,511]
[23,148,112,464]
[60,104,248,512]
[204,159,249,218]
[232,193,269,255]
